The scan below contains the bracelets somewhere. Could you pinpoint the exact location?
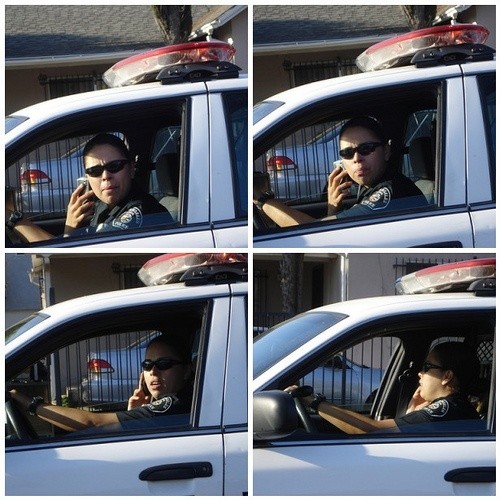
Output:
[256,191,275,210]
[309,393,327,412]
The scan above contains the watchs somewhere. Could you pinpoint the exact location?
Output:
[7,212,25,227]
[27,393,43,417]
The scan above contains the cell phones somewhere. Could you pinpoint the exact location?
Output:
[333,161,351,189]
[141,380,150,396]
[77,177,89,203]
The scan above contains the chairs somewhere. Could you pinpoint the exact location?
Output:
[155,153,179,224]
[409,137,436,205]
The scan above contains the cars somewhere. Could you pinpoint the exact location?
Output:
[17,122,128,214]
[254,323,388,415]
[263,111,420,199]
[5,39,248,249]
[253,257,496,497]
[80,320,195,411]
[6,254,248,495]
[252,24,496,248]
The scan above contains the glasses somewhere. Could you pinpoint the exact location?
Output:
[85,159,130,177]
[142,357,182,371]
[422,360,442,373]
[339,142,386,159]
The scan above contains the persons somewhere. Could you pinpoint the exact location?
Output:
[5,134,174,244]
[11,334,192,432]
[284,342,484,435]
[253,115,428,229]
